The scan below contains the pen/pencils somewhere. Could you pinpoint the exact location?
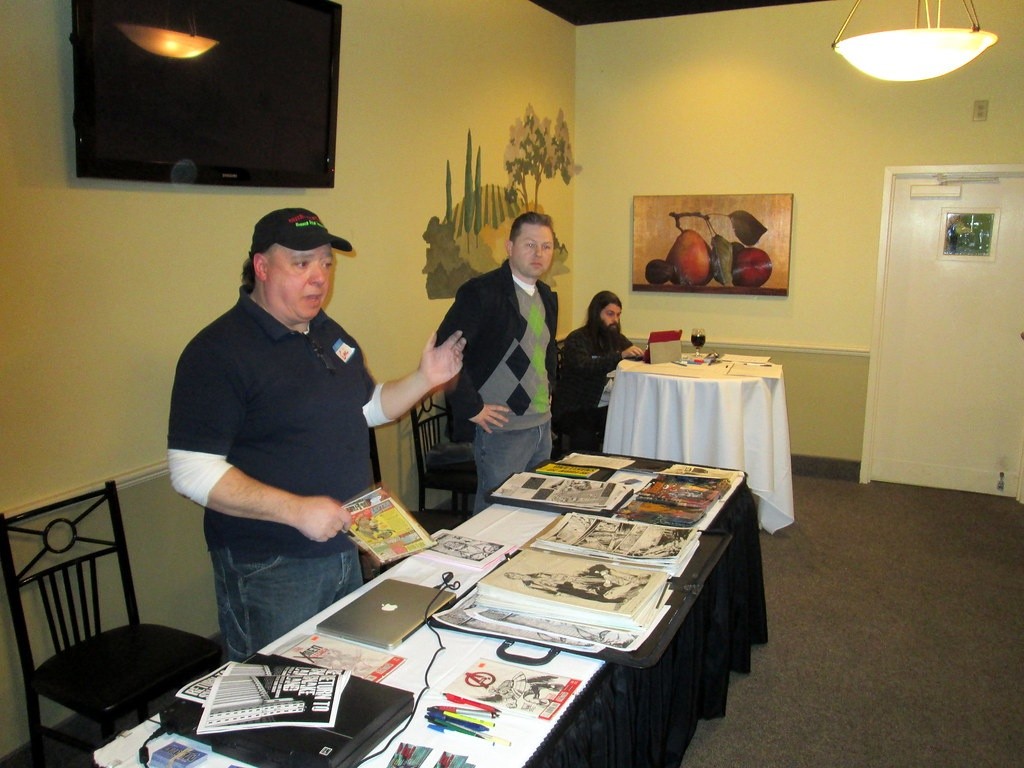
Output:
[424,705,512,747]
[443,693,502,712]
[744,364,772,367]
[671,350,715,368]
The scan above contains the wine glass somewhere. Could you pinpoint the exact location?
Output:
[691,328,706,358]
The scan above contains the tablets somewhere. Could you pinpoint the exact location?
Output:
[644,329,682,363]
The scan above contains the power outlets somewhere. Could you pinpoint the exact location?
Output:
[973,100,988,120]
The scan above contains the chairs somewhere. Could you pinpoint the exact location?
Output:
[1,480,250,768]
[553,338,566,450]
[411,382,478,522]
[359,427,463,584]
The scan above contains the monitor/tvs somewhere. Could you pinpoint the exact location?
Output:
[68,0,342,189]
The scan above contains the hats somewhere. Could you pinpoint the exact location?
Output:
[252,207,352,252]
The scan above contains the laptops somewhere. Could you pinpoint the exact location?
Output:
[315,579,456,651]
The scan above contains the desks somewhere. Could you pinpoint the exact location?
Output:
[603,352,796,535]
[92,453,768,768]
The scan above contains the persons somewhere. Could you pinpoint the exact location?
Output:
[166,207,467,667]
[434,211,558,516]
[551,291,645,456]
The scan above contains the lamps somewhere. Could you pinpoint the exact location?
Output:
[112,0,221,59]
[832,0,998,82]
